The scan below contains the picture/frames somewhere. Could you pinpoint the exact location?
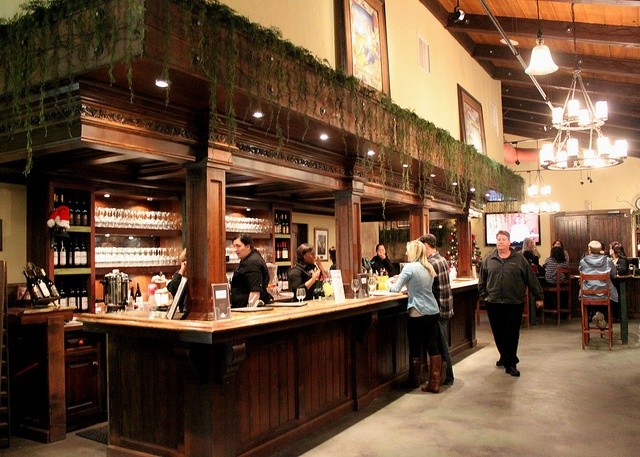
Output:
[333,0,392,100]
[314,226,330,262]
[457,83,488,157]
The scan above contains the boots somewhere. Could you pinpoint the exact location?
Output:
[421,355,442,392]
[406,356,420,387]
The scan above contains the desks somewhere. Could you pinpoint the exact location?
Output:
[7,302,76,443]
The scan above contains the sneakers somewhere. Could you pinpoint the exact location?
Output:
[497,360,505,365]
[505,364,520,376]
[593,315,608,328]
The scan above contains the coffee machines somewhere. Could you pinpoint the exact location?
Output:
[100,269,132,307]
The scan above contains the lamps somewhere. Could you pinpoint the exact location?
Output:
[539,1,628,172]
[525,1,559,76]
[520,172,561,216]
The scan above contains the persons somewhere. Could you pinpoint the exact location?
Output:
[510,215,530,243]
[542,247,568,288]
[287,243,329,302]
[479,231,545,377]
[167,248,187,314]
[387,240,442,394]
[579,241,618,329]
[522,238,546,277]
[370,244,395,278]
[552,239,570,265]
[609,242,629,276]
[229,234,274,308]
[419,234,454,387]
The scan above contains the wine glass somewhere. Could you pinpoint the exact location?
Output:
[226,247,268,264]
[296,289,303,302]
[301,288,306,302]
[350,278,359,299]
[95,207,177,230]
[368,277,377,298]
[225,216,273,234]
[95,247,178,266]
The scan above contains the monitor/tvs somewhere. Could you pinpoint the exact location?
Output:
[484,211,541,247]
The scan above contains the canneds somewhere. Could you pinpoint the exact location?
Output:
[95,300,105,315]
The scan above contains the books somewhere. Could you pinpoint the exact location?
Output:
[266,302,307,308]
[231,308,274,312]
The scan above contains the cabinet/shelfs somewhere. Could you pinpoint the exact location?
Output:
[272,208,293,291]
[92,172,275,312]
[27,168,95,329]
[61,324,106,433]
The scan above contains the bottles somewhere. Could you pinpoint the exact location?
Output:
[68,238,73,267]
[68,200,75,225]
[74,201,82,226]
[54,242,59,268]
[59,281,68,310]
[68,288,78,312]
[59,239,66,267]
[280,213,285,234]
[65,239,68,266]
[81,280,89,312]
[40,268,59,296]
[53,193,64,209]
[32,269,51,298]
[129,280,134,300]
[23,270,43,300]
[274,213,278,234]
[278,218,281,234]
[135,283,143,305]
[82,202,88,226]
[73,237,81,267]
[275,241,289,262]
[277,268,289,290]
[284,213,289,234]
[80,237,88,267]
[75,288,82,312]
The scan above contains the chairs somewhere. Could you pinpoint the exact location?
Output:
[521,285,533,331]
[541,265,574,326]
[579,270,614,351]
[477,299,489,329]
